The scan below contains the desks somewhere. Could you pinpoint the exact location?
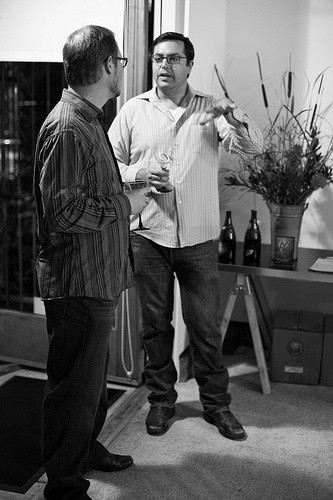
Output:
[218,241,333,394]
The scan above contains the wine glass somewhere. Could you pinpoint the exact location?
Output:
[150,143,176,194]
[126,179,151,230]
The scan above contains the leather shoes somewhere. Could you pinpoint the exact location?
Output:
[146,406,175,435]
[203,411,245,439]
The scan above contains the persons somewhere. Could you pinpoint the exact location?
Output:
[34,24,152,500]
[106,32,263,440]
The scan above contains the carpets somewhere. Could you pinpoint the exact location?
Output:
[0,375,127,494]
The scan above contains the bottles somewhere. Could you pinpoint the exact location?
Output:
[217,211,237,264]
[244,209,262,266]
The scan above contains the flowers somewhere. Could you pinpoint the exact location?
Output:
[213,52,333,205]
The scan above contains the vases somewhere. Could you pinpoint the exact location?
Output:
[268,205,305,269]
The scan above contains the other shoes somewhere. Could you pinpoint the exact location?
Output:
[93,453,133,472]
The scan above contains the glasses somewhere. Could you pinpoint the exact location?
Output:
[103,56,128,68]
[151,54,187,64]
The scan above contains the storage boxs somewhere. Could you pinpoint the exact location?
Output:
[271,310,333,387]
